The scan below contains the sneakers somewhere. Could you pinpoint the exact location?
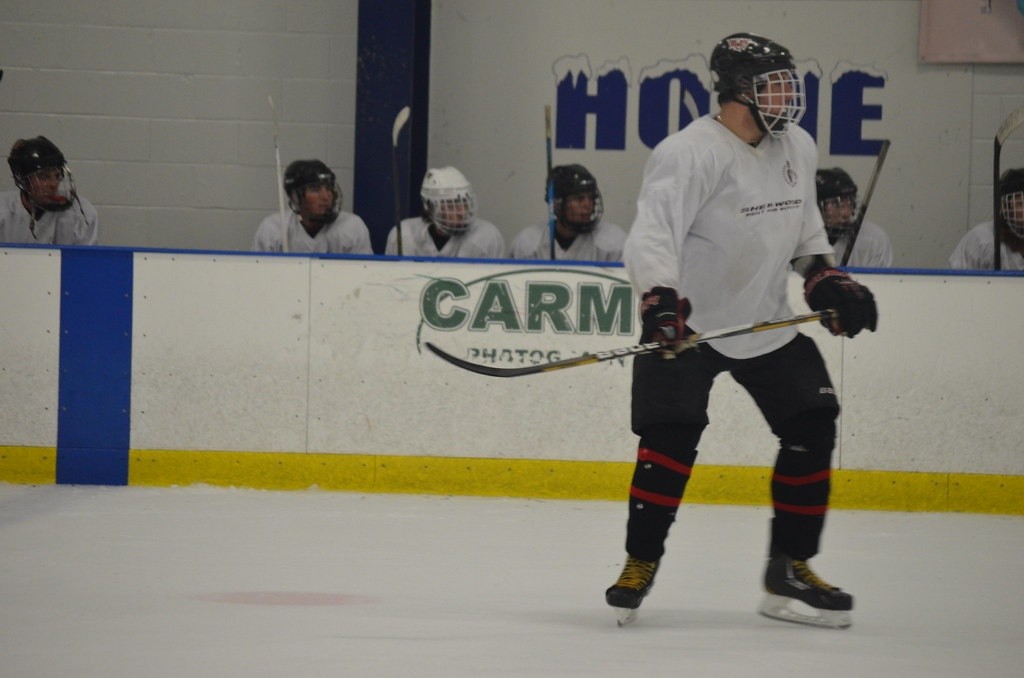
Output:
[605,554,660,626]
[760,552,855,628]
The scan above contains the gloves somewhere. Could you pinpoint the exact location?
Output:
[802,268,878,339]
[641,287,693,359]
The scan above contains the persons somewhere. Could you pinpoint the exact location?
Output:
[815,167,892,267]
[0,136,100,246]
[253,158,373,254]
[605,33,879,628]
[384,165,506,259]
[948,167,1024,270]
[507,162,628,262]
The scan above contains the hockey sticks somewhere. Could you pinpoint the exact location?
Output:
[266,92,288,253]
[994,105,1024,271]
[392,107,417,255]
[840,140,891,267]
[543,102,556,257]
[425,308,840,377]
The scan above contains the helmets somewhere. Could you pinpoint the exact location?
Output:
[6,135,77,213]
[420,165,478,236]
[813,167,857,247]
[282,158,343,225]
[996,166,1024,253]
[544,164,605,235]
[709,33,806,139]
